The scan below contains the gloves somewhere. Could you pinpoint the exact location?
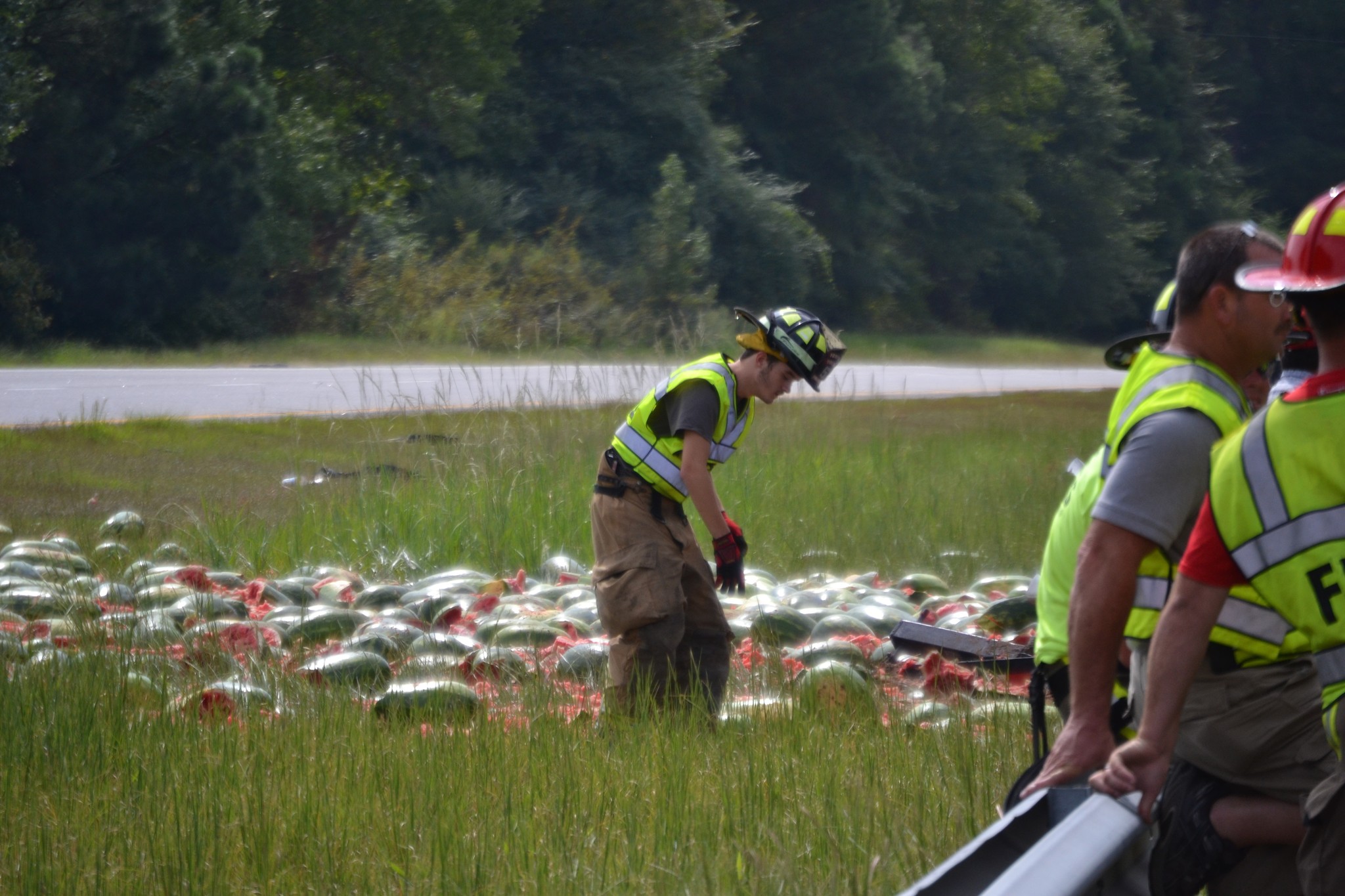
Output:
[712,532,747,598]
[721,510,748,557]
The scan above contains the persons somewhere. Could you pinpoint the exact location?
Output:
[591,308,845,721]
[1018,186,1345,895]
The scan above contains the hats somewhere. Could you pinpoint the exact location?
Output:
[1232,179,1345,292]
[1104,276,1178,370]
[733,304,845,393]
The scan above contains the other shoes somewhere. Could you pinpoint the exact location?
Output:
[1149,758,1250,896]
